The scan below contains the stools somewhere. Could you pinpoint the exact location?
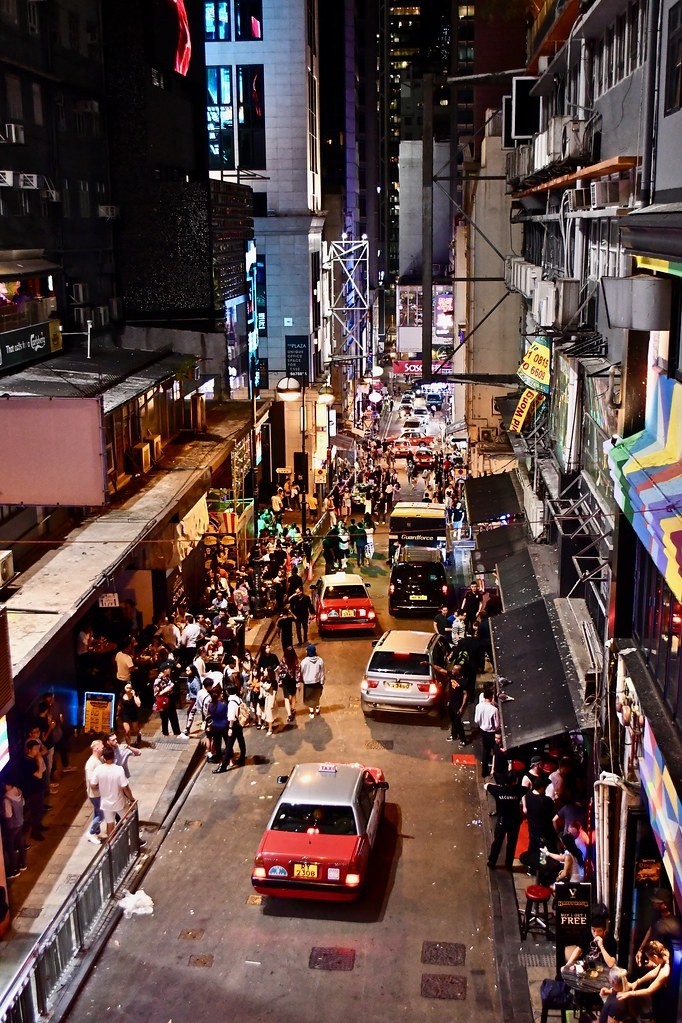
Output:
[520,884,552,942]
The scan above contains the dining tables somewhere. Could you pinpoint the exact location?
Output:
[234,510,304,609]
[560,959,614,1023]
[131,585,249,693]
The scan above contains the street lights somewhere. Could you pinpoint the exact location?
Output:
[299,378,336,538]
[353,364,383,429]
[250,364,303,541]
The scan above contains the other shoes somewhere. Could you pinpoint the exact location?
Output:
[49,783,59,793]
[309,706,320,718]
[446,736,453,742]
[136,720,272,773]
[30,825,48,841]
[62,766,78,772]
[287,709,296,721]
[41,804,53,814]
[458,742,465,749]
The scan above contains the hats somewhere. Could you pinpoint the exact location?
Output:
[306,645,316,651]
[530,756,543,767]
[160,661,173,668]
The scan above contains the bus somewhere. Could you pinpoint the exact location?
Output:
[388,501,451,559]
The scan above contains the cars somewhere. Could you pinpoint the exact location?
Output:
[380,354,451,411]
[413,447,435,468]
[390,439,413,457]
[309,572,376,636]
[385,431,434,448]
[409,406,430,425]
[385,543,443,571]
[402,418,426,433]
[250,759,391,903]
[388,561,449,618]
[399,404,413,417]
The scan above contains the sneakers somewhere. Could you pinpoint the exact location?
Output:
[16,843,31,853]
[6,866,27,878]
[88,833,101,844]
[139,839,147,848]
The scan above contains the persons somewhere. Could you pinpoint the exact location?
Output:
[599,940,672,1023]
[635,888,675,968]
[84,739,109,845]
[100,731,142,829]
[0,692,78,879]
[89,746,147,849]
[597,964,629,1023]
[114,432,501,777]
[75,626,98,675]
[484,745,591,915]
[558,914,618,970]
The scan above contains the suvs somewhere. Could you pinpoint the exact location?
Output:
[359,629,455,719]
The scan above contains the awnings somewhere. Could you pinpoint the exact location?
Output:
[477,518,533,573]
[494,543,562,612]
[463,467,527,525]
[483,596,600,753]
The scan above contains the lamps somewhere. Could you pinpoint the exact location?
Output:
[318,386,335,402]
[276,376,302,401]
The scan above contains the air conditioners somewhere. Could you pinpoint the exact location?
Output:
[0,171,21,188]
[0,549,15,588]
[143,434,162,461]
[17,174,45,191]
[132,442,151,473]
[191,392,207,433]
[5,123,25,144]
[504,114,574,181]
[41,189,60,202]
[566,188,591,210]
[590,180,633,209]
[560,119,588,161]
[98,205,117,218]
[504,254,543,299]
[531,280,556,327]
